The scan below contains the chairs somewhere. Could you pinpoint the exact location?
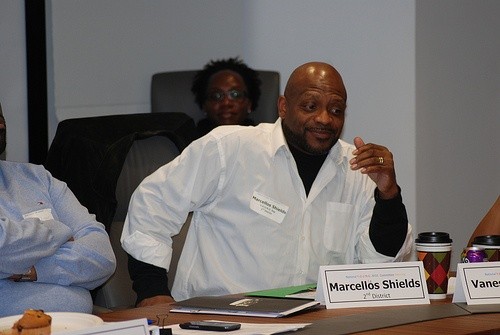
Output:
[45,112,196,311]
[151,70,280,125]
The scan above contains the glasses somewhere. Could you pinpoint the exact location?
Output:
[205,90,249,102]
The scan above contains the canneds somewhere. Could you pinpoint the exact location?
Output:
[460,247,488,263]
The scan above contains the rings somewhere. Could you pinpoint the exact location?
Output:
[379,157,383,165]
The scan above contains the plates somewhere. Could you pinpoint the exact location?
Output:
[0,311,104,335]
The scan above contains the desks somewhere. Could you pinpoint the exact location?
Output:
[96,271,500,335]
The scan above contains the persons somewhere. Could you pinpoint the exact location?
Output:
[120,62,414,308]
[0,103,116,319]
[191,56,260,138]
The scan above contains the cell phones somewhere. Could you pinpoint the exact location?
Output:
[179,320,241,332]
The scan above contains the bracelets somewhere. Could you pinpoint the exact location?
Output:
[13,269,31,282]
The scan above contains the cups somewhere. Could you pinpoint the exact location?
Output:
[472,234,500,262]
[416,231,452,300]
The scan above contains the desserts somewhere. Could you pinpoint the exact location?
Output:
[12,309,52,335]
